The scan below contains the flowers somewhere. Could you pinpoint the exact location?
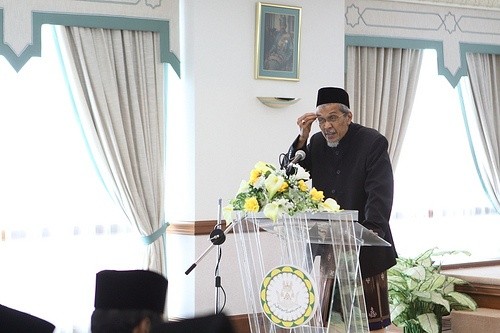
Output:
[224,161,345,222]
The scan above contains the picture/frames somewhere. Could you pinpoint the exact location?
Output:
[254,2,300,82]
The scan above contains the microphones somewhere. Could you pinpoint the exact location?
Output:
[284,150,306,171]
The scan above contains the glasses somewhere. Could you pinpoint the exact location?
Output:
[315,113,345,123]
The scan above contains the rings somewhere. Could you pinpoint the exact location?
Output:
[302,120,306,125]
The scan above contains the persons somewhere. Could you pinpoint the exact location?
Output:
[281,87,391,333]
[90,270,168,333]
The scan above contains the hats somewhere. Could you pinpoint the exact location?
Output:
[94,270,168,313]
[316,87,349,109]
